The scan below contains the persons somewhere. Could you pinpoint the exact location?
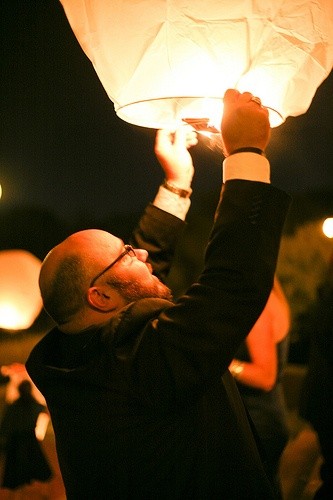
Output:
[0,90,333,500]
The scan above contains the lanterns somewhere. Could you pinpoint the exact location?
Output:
[60,1,332,135]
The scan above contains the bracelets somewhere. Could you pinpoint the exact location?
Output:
[228,146,267,158]
[233,361,243,382]
[164,179,193,198]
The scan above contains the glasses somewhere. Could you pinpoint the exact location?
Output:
[89,245,137,287]
